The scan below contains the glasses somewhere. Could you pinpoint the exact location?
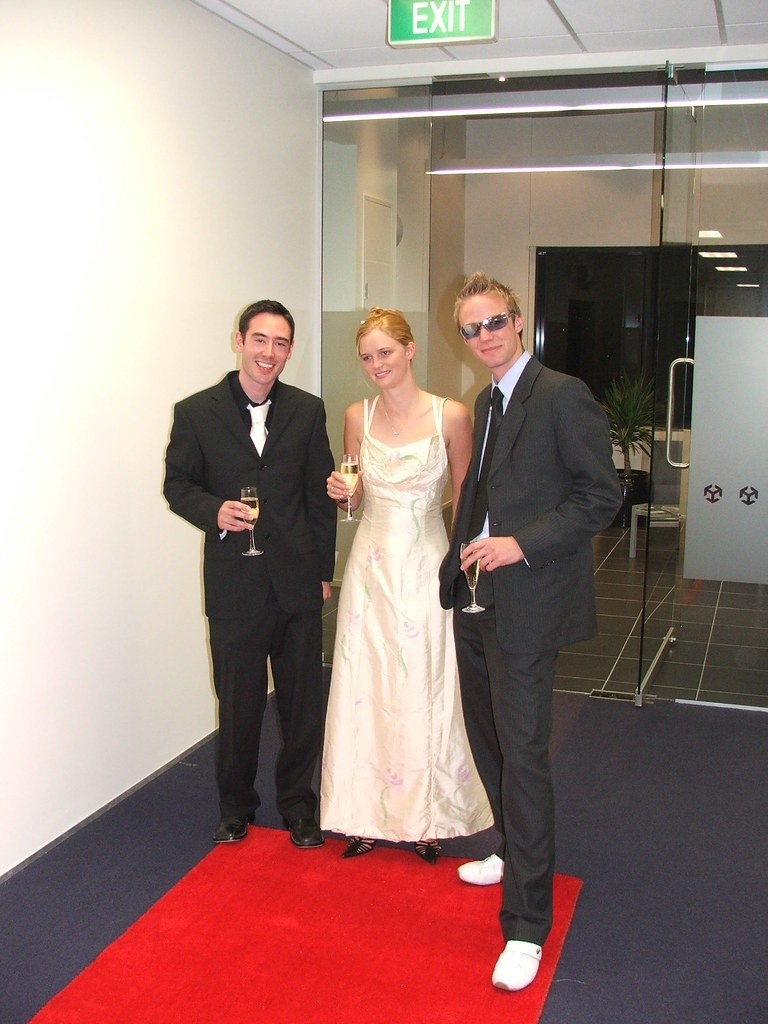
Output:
[460,311,514,340]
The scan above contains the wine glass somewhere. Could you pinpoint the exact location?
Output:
[338,453,361,522]
[240,487,264,555]
[460,543,485,613]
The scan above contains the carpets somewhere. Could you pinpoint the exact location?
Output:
[25,825,584,1024]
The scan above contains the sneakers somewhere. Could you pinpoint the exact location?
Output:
[457,853,505,885]
[492,939,542,991]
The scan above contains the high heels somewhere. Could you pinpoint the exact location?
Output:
[414,839,442,865]
[342,836,378,858]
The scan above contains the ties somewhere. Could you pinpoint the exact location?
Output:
[246,399,272,457]
[466,387,504,542]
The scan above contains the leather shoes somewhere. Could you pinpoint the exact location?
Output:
[282,816,325,848]
[212,810,255,842]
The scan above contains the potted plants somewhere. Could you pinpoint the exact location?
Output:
[594,370,664,528]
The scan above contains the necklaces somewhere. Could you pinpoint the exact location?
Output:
[381,386,421,436]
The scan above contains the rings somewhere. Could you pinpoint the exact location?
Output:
[331,487,334,492]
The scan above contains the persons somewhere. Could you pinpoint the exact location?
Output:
[438,268,624,992]
[163,299,340,849]
[327,308,478,865]
